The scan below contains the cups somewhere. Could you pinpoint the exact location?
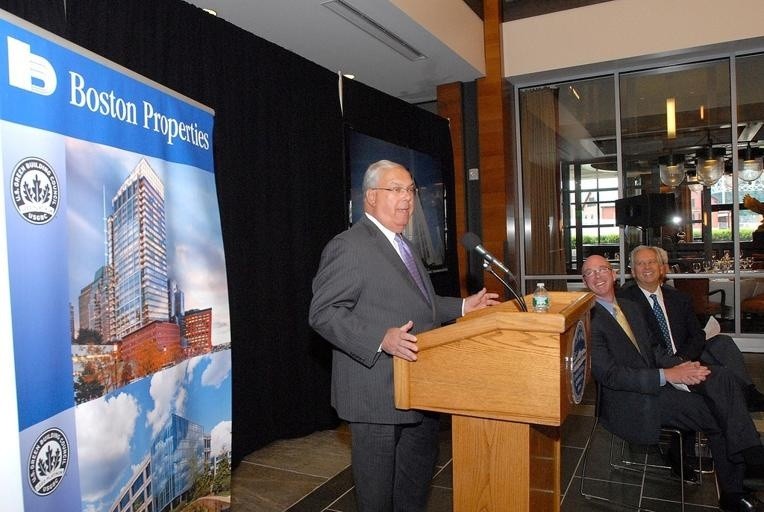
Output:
[603,251,609,258]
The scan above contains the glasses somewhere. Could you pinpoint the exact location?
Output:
[373,186,419,195]
[582,266,611,277]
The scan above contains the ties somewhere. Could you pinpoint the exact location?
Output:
[650,294,674,355]
[395,234,429,299]
[613,304,639,350]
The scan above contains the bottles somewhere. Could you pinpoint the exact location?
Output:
[530,283,549,314]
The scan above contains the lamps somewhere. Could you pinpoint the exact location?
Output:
[658,121,763,189]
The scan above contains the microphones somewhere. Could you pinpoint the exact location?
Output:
[461,229,528,312]
[480,261,526,312]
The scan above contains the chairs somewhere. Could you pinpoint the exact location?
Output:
[559,374,716,512]
[696,249,745,260]
[673,276,731,332]
[739,291,764,334]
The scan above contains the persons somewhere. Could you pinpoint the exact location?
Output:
[582,255,764,512]
[308,160,502,512]
[618,246,764,414]
[619,246,675,290]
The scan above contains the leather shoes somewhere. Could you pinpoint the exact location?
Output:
[718,494,763,511]
[748,391,764,412]
[741,457,763,488]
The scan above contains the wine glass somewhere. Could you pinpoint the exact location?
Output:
[692,251,754,274]
